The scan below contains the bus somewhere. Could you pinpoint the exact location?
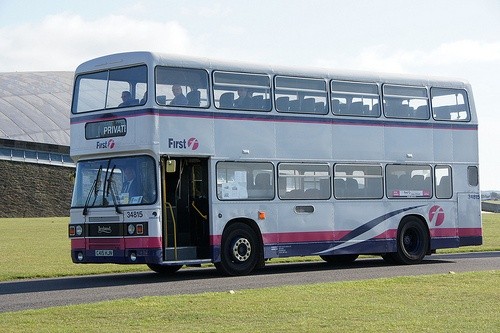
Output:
[66,49,484,278]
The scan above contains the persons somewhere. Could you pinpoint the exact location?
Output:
[121,165,144,198]
[233,88,255,108]
[119,91,139,107]
[170,84,188,104]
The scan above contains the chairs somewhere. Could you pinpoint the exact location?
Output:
[247,171,450,198]
[133,92,452,121]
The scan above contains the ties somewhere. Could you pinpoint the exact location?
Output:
[125,183,129,192]
